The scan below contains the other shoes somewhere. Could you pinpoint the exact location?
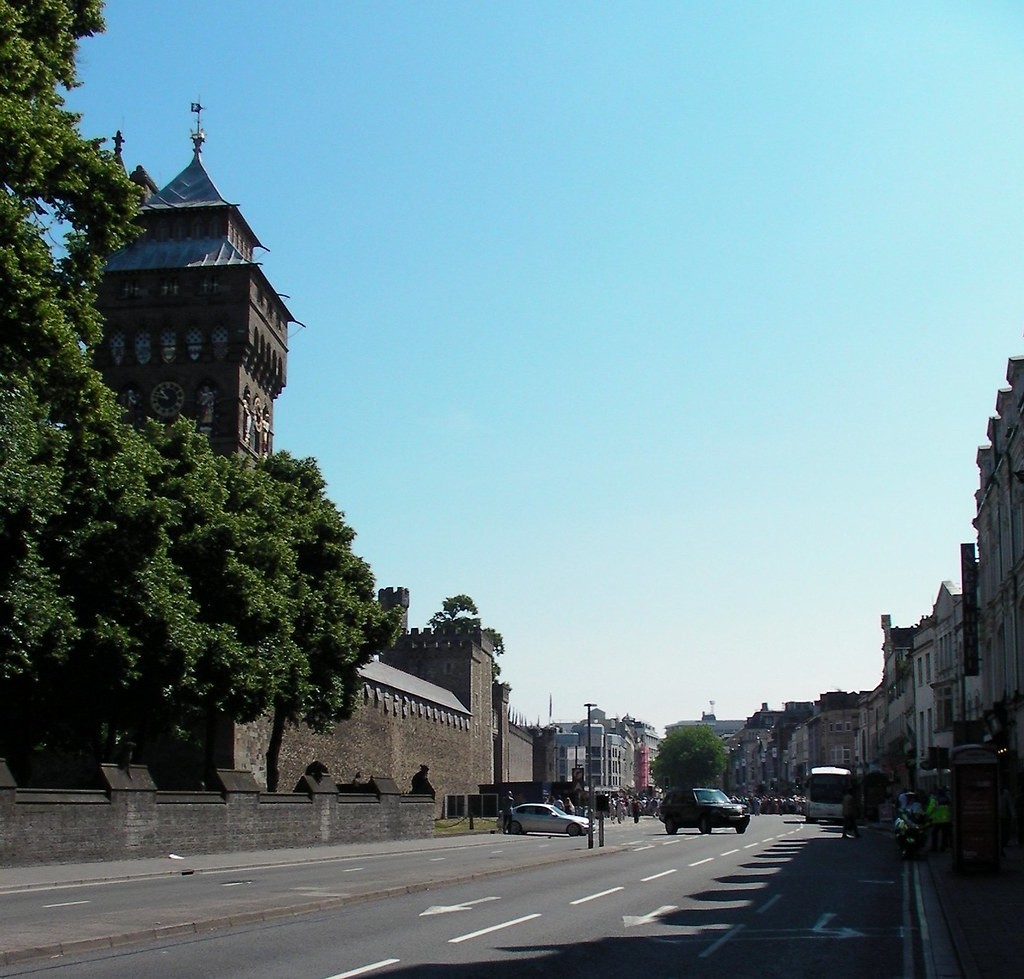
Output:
[635,820,639,823]
[842,836,849,839]
[941,846,945,852]
[1000,851,1006,858]
[855,834,862,838]
[1005,845,1012,847]
[928,846,937,851]
[503,831,511,834]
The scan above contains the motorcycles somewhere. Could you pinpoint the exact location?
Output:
[894,808,931,859]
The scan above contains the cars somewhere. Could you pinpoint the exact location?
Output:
[496,804,595,837]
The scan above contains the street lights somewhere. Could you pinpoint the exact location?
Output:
[584,704,598,849]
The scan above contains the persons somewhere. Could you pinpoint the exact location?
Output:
[729,793,807,816]
[502,790,514,834]
[999,782,1024,857]
[354,771,365,786]
[926,783,953,854]
[543,793,662,823]
[841,786,861,839]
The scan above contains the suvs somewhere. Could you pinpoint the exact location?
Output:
[657,787,750,834]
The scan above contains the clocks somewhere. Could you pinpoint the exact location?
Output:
[148,379,185,418]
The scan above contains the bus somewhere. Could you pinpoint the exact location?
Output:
[805,766,852,825]
